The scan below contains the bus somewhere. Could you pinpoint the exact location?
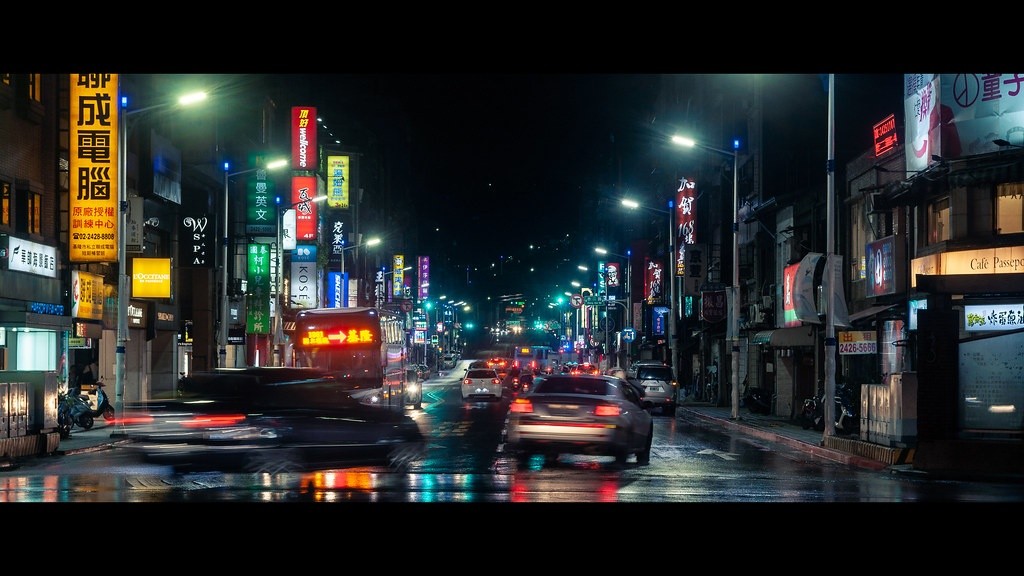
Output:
[293,306,408,415]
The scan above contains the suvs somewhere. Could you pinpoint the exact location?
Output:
[627,364,679,414]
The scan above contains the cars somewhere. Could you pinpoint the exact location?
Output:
[406,364,431,409]
[487,346,601,394]
[458,368,504,401]
[102,367,425,475]
[506,374,654,466]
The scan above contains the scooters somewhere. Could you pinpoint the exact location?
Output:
[54,376,114,439]
[800,379,860,436]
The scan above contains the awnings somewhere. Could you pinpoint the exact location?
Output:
[752,326,815,348]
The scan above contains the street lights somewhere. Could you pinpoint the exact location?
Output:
[341,237,381,307]
[217,158,290,368]
[577,266,608,370]
[433,295,470,373]
[272,195,331,368]
[570,280,586,363]
[564,291,580,344]
[593,245,632,368]
[108,87,209,439]
[376,265,413,308]
[671,134,743,421]
